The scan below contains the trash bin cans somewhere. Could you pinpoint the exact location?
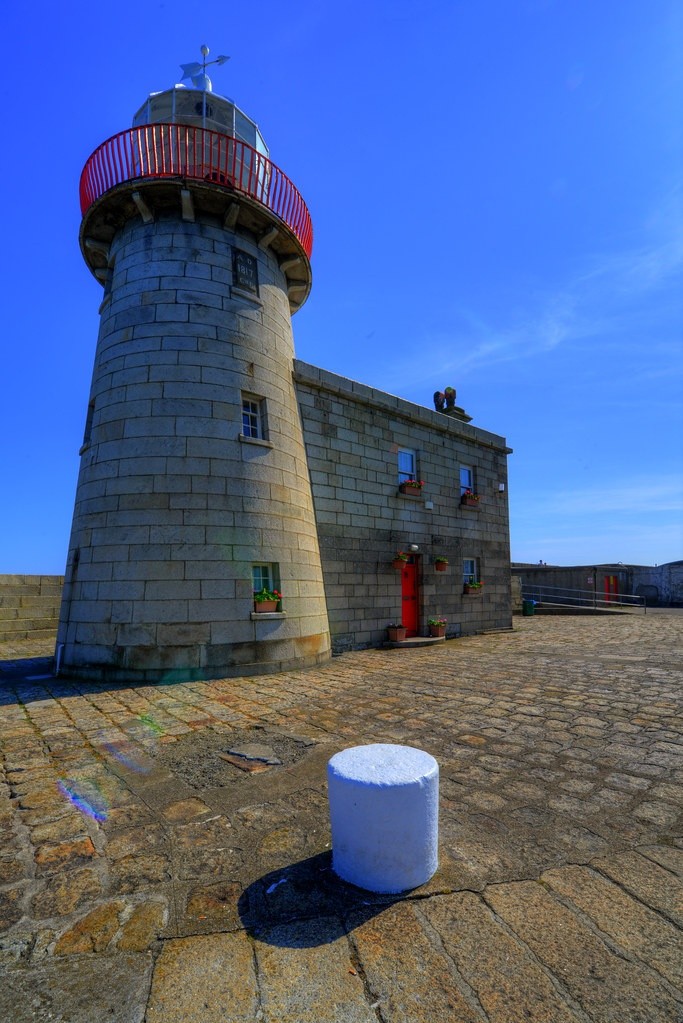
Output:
[523,600,534,616]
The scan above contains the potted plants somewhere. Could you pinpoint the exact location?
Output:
[385,625,408,641]
[433,555,449,571]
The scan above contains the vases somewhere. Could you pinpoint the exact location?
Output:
[430,625,446,637]
[399,486,422,496]
[461,498,479,507]
[467,587,480,594]
[255,601,278,612]
[394,560,407,570]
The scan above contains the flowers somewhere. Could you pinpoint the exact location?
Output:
[462,490,481,500]
[427,616,448,627]
[254,588,282,602]
[400,480,425,487]
[391,550,409,561]
[466,580,485,588]
